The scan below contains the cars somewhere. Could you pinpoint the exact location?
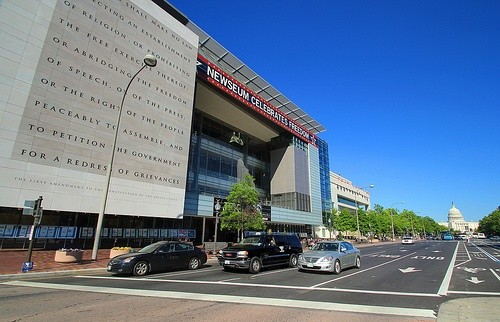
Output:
[402,237,415,244]
[298,241,362,275]
[106,240,209,277]
[489,236,500,241]
[455,232,486,240]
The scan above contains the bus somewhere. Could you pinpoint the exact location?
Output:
[441,231,452,241]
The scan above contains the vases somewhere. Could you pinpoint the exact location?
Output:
[55,249,84,264]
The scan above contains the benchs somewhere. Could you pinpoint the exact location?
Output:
[203,242,227,255]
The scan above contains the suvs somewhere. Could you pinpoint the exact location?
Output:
[216,232,304,275]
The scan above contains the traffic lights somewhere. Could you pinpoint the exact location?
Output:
[22,198,41,219]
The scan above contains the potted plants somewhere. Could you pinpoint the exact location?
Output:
[109,246,132,259]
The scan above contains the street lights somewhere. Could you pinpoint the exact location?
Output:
[391,201,406,242]
[410,211,421,238]
[91,53,158,263]
[355,185,375,243]
[214,198,221,257]
[328,218,333,243]
